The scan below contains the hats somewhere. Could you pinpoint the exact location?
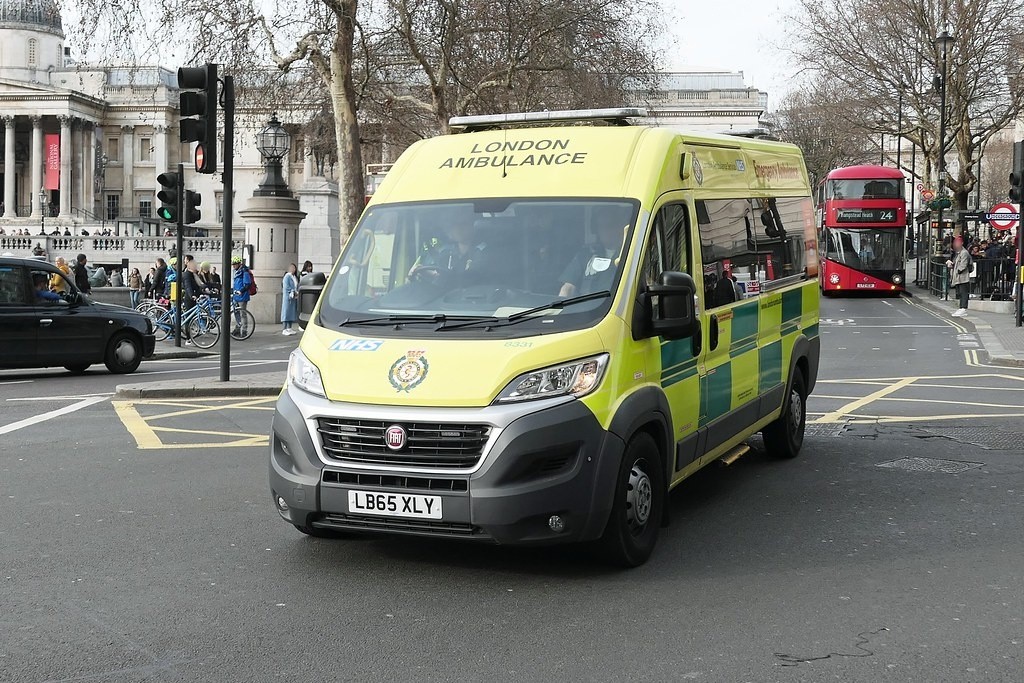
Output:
[77,254,86,260]
[187,260,197,270]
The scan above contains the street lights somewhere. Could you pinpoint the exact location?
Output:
[876,119,888,166]
[101,151,109,232]
[39,185,47,236]
[933,31,955,257]
[896,83,910,167]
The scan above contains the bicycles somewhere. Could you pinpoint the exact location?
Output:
[194,290,255,341]
[134,290,209,336]
[142,295,220,350]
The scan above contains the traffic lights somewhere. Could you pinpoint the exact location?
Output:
[157,172,178,223]
[177,63,218,174]
[1008,140,1024,204]
[183,189,201,224]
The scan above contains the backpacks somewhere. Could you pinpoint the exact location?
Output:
[249,271,258,295]
[967,255,974,273]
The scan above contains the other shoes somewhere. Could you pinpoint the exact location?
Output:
[282,328,297,336]
[231,329,240,337]
[242,330,247,337]
[185,339,197,346]
[951,308,968,318]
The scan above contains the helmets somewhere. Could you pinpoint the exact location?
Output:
[168,257,177,265]
[231,256,241,265]
[200,261,209,270]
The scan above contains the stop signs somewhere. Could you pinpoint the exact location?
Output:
[196,145,204,169]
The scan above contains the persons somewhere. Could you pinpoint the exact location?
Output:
[946,238,970,317]
[32,242,45,256]
[0,228,31,249]
[943,232,1018,298]
[39,226,71,250]
[705,271,743,310]
[281,260,313,335]
[137,228,144,249]
[32,253,93,305]
[107,254,251,345]
[81,228,112,250]
[194,228,205,251]
[859,239,876,264]
[558,205,631,300]
[163,227,178,237]
[404,208,493,292]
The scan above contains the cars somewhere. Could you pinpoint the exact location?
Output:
[0,257,155,373]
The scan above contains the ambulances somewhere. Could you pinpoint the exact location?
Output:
[268,106,820,571]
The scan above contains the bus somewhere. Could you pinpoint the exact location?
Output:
[812,165,906,296]
